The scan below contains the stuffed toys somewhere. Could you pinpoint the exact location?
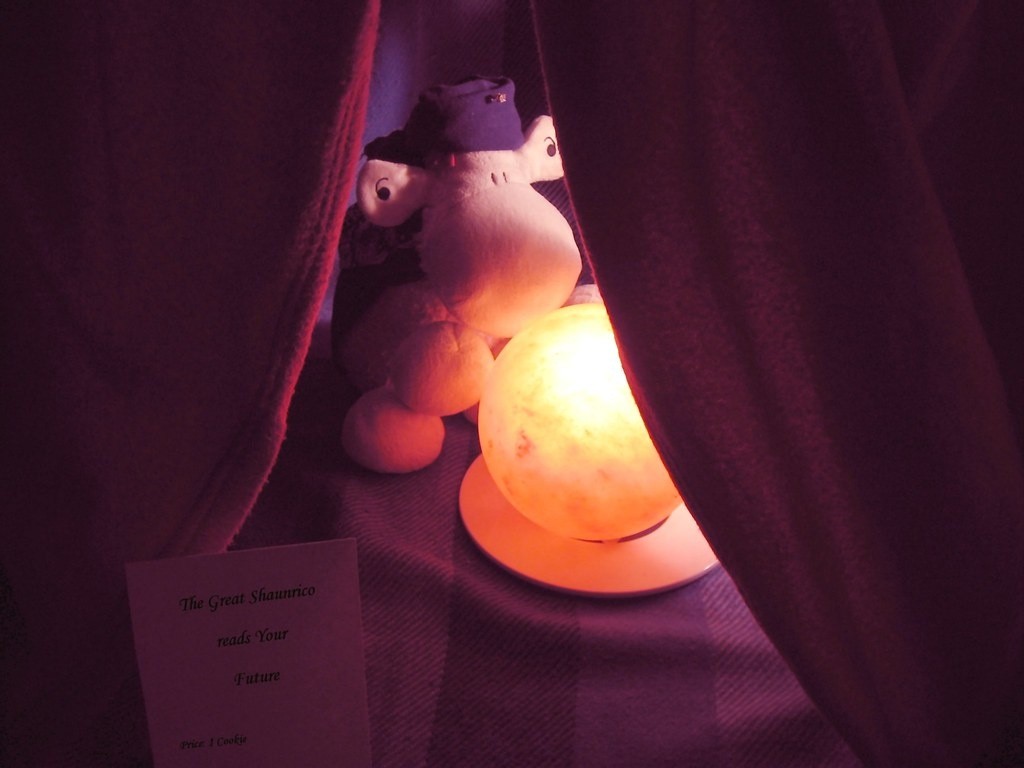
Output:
[332,73,582,473]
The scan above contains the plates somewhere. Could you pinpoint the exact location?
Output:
[459,454,721,599]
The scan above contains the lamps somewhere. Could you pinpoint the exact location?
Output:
[476,303,683,544]
